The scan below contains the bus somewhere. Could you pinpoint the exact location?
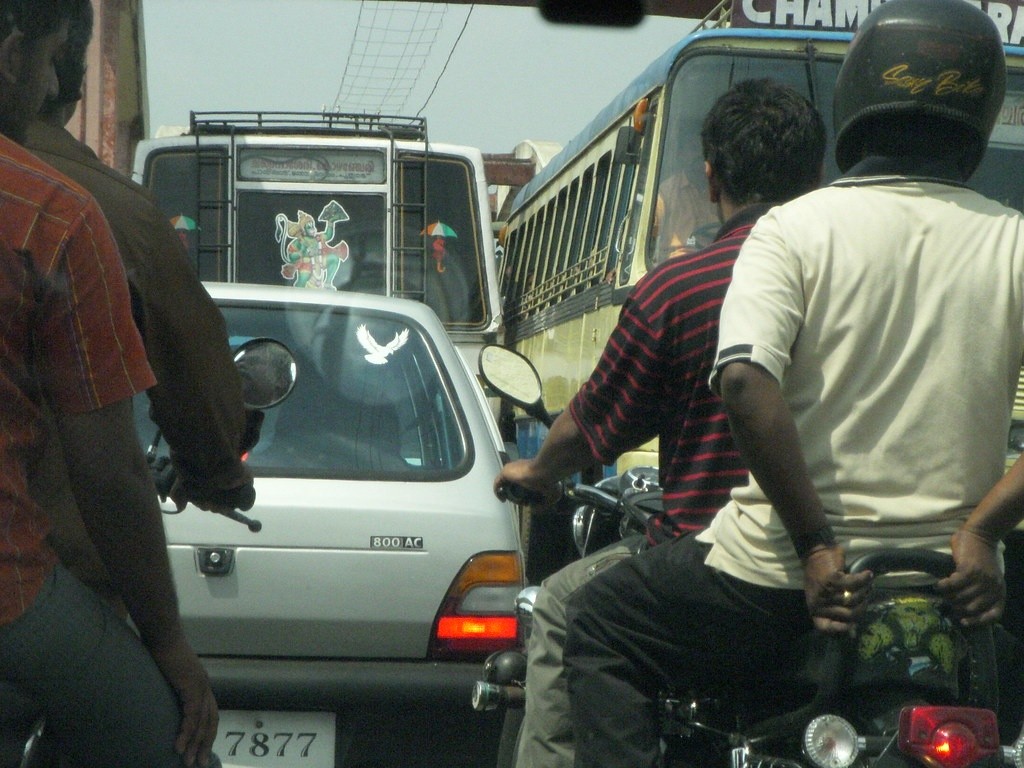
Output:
[126,110,507,426]
[489,29,1024,746]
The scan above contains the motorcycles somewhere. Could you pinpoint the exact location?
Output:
[471,343,1006,768]
[1,337,296,767]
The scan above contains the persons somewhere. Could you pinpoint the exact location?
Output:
[0,2,253,768]
[494,80,820,768]
[562,0,1023,768]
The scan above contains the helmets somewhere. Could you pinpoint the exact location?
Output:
[827,0,1008,183]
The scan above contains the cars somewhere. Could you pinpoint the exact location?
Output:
[134,282,529,768]
